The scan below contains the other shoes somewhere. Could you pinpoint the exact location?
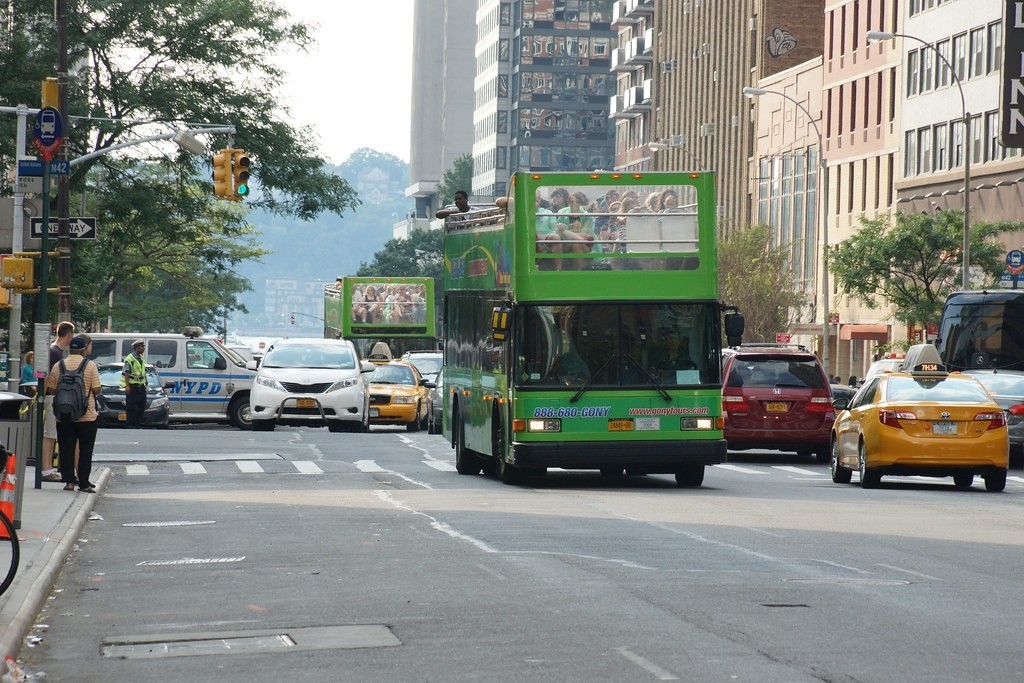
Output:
[88,482,95,488]
[42,473,62,481]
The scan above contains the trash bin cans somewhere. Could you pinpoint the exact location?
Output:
[0,391,30,529]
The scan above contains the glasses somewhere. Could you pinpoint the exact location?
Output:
[612,208,619,211]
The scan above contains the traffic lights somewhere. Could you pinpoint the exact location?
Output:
[233,153,251,196]
[211,149,233,196]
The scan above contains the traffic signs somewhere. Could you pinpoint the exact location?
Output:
[29,216,96,240]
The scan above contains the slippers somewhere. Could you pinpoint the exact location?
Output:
[63,484,74,490]
[81,487,96,493]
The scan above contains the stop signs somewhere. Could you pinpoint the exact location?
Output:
[258,342,266,348]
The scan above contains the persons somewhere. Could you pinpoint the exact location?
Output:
[648,328,697,379]
[48,334,101,494]
[332,278,343,288]
[849,376,857,387]
[23,351,38,382]
[119,339,148,429]
[436,191,481,227]
[352,284,426,323]
[494,188,686,269]
[830,375,841,384]
[43,321,74,482]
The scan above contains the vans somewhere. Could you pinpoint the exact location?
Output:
[866,352,907,383]
[63,326,265,430]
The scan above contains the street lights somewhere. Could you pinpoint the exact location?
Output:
[866,31,971,291]
[743,87,832,383]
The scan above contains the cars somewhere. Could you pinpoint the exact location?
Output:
[247,339,376,435]
[94,362,170,429]
[829,344,1011,492]
[359,342,443,434]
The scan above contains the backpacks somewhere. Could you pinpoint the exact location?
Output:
[52,359,91,421]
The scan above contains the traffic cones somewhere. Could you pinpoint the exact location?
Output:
[0,453,27,541]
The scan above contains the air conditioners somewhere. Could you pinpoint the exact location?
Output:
[662,62,670,73]
[703,44,710,54]
[732,116,738,126]
[695,49,699,59]
[713,71,717,80]
[694,0,700,7]
[701,124,714,136]
[670,135,680,146]
[749,18,756,30]
[671,59,677,68]
[683,3,690,13]
[660,139,669,149]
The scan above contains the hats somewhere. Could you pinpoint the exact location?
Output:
[70,337,86,348]
[131,338,146,348]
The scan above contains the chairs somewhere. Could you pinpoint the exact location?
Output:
[662,208,700,254]
[626,208,663,253]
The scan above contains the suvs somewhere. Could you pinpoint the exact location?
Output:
[722,342,837,464]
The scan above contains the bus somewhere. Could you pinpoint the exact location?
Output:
[931,289,1024,468]
[441,170,745,488]
